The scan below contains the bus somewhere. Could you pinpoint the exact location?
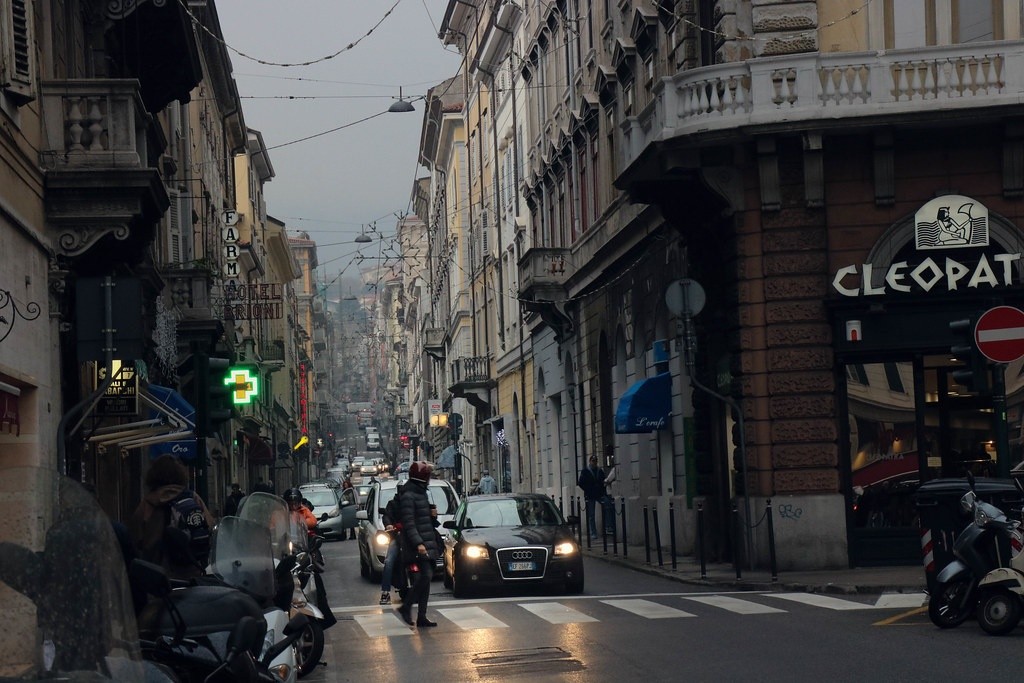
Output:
[357,412,372,429]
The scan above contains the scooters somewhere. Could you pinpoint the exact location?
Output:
[928,469,1024,636]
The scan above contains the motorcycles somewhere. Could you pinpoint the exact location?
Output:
[114,492,330,683]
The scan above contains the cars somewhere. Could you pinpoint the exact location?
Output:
[299,445,413,541]
[355,478,463,583]
[443,491,585,598]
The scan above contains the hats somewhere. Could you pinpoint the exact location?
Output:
[590,455,597,460]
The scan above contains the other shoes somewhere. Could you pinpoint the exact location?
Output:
[592,533,598,539]
[416,617,437,627]
[380,593,391,605]
[398,605,415,626]
[349,536,356,540]
[606,529,614,534]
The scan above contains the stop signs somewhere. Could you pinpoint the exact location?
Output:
[974,306,1024,362]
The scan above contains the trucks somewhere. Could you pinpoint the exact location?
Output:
[365,426,381,451]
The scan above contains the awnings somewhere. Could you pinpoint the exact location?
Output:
[153,382,199,462]
[615,377,670,435]
[435,445,457,470]
[238,428,273,462]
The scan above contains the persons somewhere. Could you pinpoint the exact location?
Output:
[370,476,381,490]
[268,486,326,564]
[379,458,445,628]
[38,476,132,673]
[224,483,244,515]
[251,476,275,494]
[343,480,359,540]
[577,453,616,538]
[136,453,217,567]
[478,469,496,494]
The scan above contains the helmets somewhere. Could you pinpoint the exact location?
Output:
[409,461,432,484]
[283,488,302,506]
[397,479,408,493]
